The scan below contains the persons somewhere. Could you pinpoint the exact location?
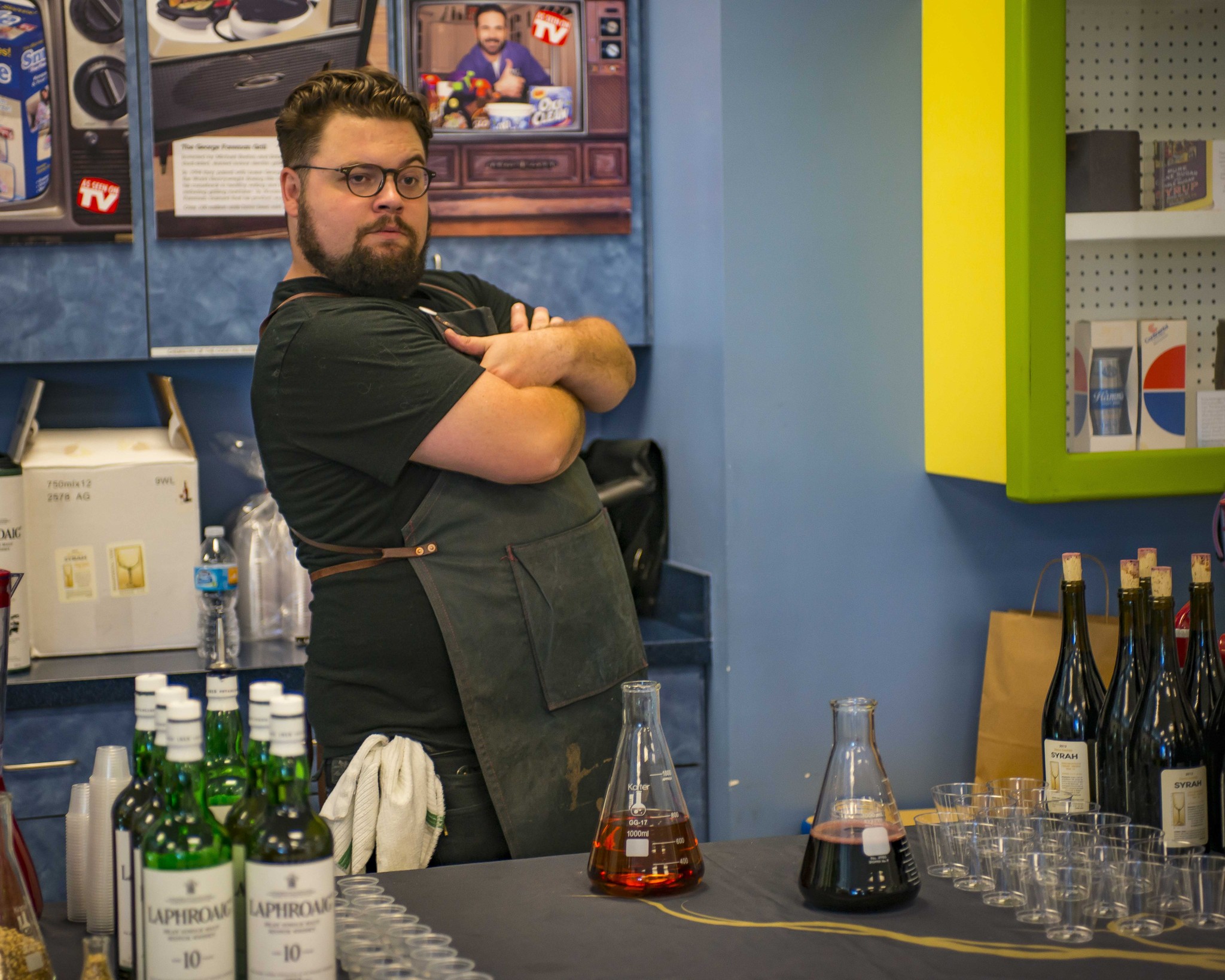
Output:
[454,4,553,105]
[35,88,51,126]
[250,64,654,868]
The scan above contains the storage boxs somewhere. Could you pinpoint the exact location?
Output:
[1153,140,1225,211]
[1073,319,1139,450]
[8,374,201,658]
[1139,321,1188,448]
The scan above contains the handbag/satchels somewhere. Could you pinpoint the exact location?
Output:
[578,438,671,613]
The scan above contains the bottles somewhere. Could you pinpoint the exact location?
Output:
[1042,547,1225,876]
[194,525,241,660]
[0,453,33,673]
[110,672,337,979]
[0,569,26,773]
[799,697,921,911]
[0,776,57,979]
[79,937,112,980]
[589,681,705,900]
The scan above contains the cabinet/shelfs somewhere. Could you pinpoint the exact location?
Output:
[3,664,705,909]
[919,0,1225,502]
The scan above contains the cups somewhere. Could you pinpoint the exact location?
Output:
[66,745,132,936]
[832,799,884,822]
[335,876,493,980]
[913,777,1225,947]
[1089,357,1123,435]
[235,503,313,646]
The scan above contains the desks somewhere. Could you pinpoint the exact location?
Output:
[336,822,1225,980]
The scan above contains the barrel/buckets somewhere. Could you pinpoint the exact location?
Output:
[485,102,535,129]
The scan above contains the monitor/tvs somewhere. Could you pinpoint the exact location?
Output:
[145,0,378,142]
[0,0,132,234]
[386,0,629,136]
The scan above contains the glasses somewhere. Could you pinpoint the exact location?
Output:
[292,164,436,199]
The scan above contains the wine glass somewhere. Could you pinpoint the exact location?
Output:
[1173,793,1184,826]
[1051,762,1059,789]
